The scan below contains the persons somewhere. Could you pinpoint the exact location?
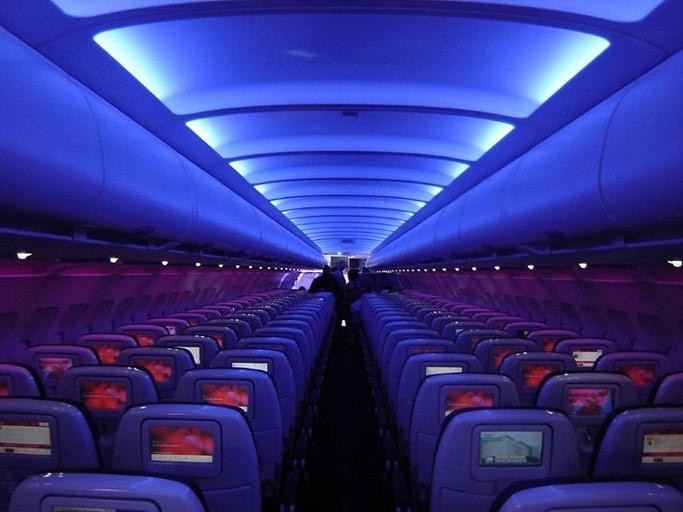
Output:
[297,258,394,311]
